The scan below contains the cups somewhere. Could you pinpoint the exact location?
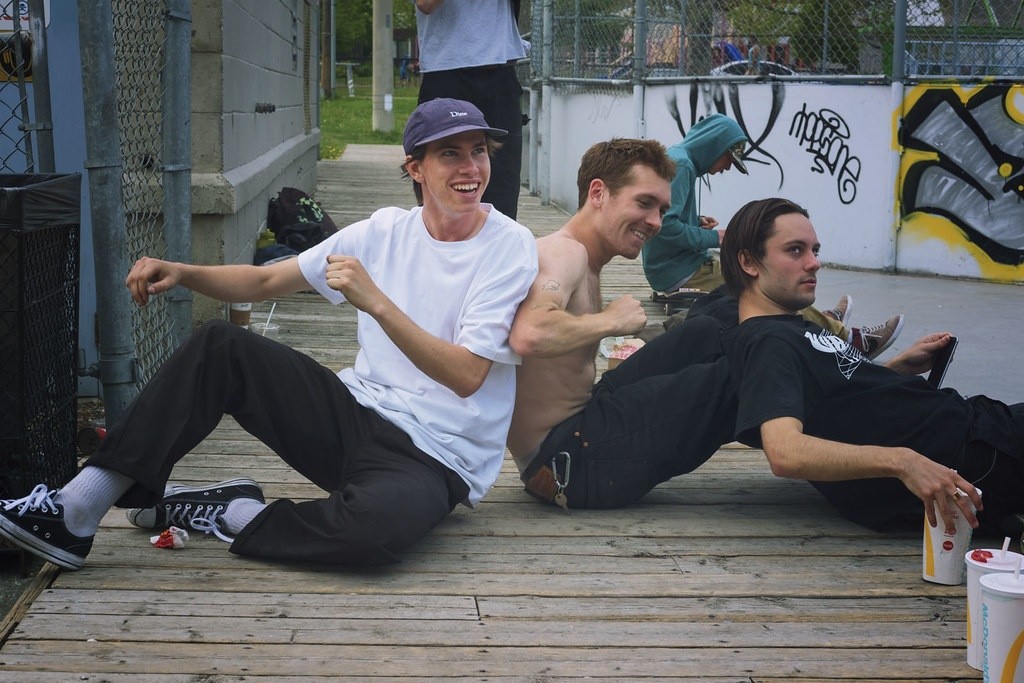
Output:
[964,547,1024,673]
[923,487,982,586]
[250,322,280,341]
[980,572,1024,682]
[229,301,252,329]
[77,427,108,454]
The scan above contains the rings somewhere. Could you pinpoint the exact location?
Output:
[950,491,963,501]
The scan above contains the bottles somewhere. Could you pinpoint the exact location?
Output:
[257,229,276,250]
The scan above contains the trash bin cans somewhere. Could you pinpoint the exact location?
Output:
[0,168,82,495]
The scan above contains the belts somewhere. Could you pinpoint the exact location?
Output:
[537,415,582,464]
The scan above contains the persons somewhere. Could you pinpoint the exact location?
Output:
[506,138,761,511]
[413,0,528,223]
[642,113,905,360]
[0,96,538,572]
[719,198,1023,534]
[399,58,420,86]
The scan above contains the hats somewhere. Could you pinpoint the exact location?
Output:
[401,97,509,154]
[728,139,748,175]
[747,32,757,40]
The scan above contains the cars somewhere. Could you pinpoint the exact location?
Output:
[710,60,823,84]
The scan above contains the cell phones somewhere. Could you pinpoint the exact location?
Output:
[927,336,960,389]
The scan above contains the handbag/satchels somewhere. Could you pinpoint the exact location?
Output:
[268,186,338,251]
[253,244,298,275]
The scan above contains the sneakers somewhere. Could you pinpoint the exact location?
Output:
[125,476,265,543]
[824,294,853,326]
[0,483,95,571]
[848,312,904,361]
